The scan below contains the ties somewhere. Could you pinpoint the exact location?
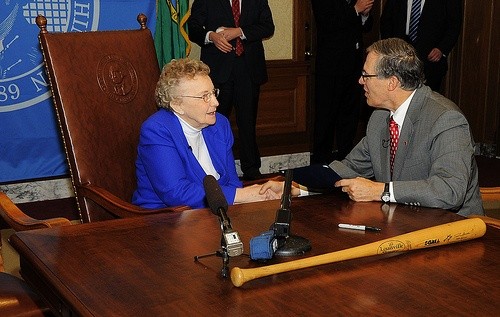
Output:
[389,116,399,181]
[409,0,421,41]
[231,0,243,56]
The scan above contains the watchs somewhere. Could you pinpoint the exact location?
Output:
[381,182,391,204]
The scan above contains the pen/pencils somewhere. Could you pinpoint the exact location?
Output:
[337,223,383,232]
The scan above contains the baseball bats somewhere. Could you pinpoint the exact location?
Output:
[230,219,488,287]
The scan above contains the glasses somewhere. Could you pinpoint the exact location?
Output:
[361,72,389,82]
[177,90,220,103]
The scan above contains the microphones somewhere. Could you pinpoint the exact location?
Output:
[202,175,232,232]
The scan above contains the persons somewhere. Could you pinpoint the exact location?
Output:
[187,0,275,181]
[130,58,281,209]
[259,38,486,221]
[307,0,465,161]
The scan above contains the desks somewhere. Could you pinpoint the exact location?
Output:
[8,192,500,317]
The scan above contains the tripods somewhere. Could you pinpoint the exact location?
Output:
[194,229,250,279]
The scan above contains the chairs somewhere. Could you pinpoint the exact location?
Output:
[0,190,69,317]
[36,12,284,222]
